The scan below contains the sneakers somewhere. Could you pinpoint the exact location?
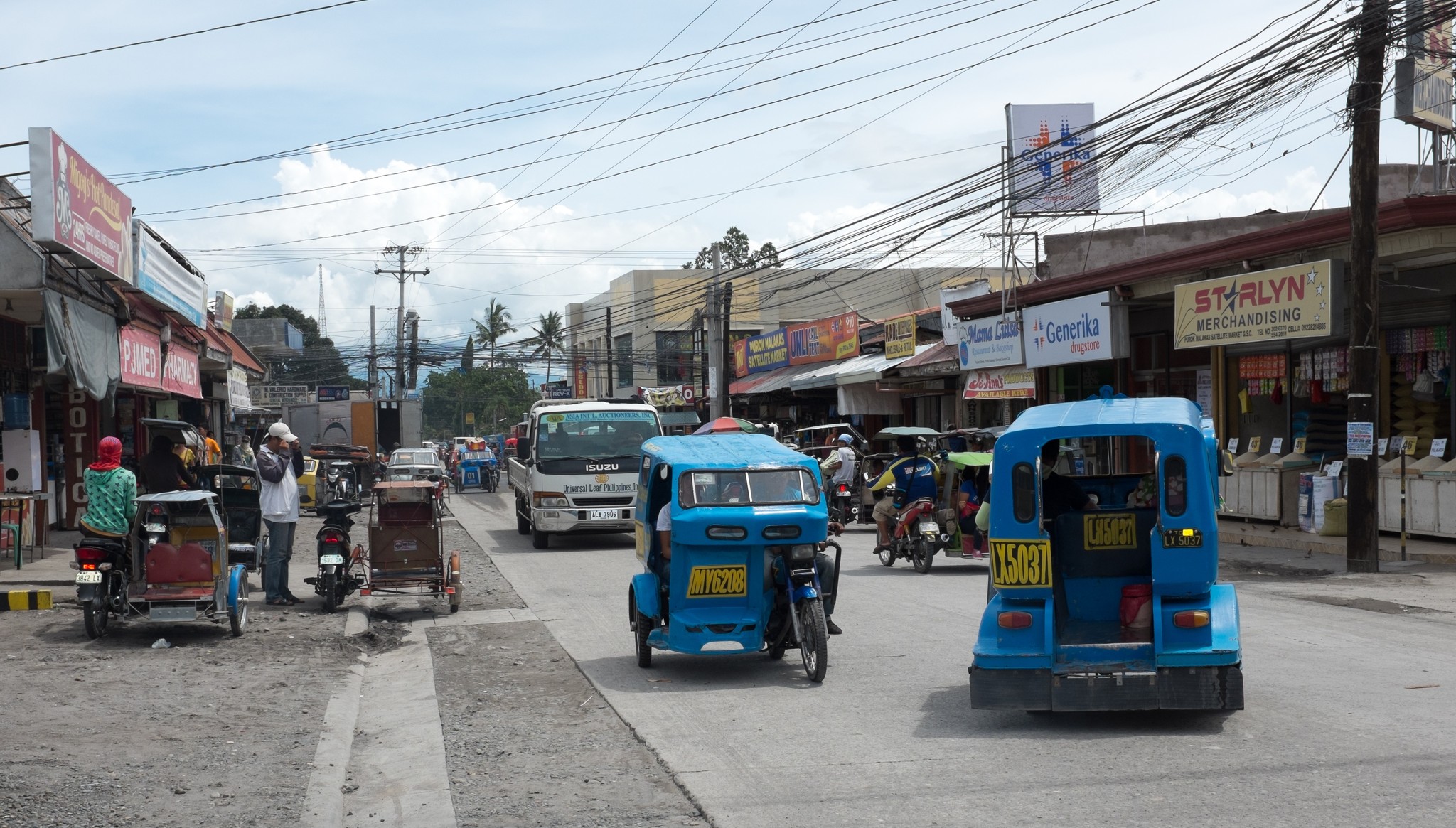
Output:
[972,549,983,560]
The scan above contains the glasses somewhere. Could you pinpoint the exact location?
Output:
[837,440,846,443]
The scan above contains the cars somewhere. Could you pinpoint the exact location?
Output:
[422,440,433,448]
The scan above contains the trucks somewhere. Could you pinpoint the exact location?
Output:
[506,396,685,549]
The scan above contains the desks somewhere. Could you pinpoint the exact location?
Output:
[0,493,53,570]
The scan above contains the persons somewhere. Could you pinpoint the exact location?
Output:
[481,467,500,489]
[256,422,305,606]
[1127,448,1187,509]
[173,420,223,488]
[444,444,455,470]
[739,472,845,634]
[423,443,427,448]
[968,438,984,453]
[140,435,197,494]
[79,436,138,584]
[656,473,715,582]
[975,439,1101,567]
[490,442,501,456]
[439,445,446,455]
[508,443,515,455]
[867,435,940,554]
[232,435,256,490]
[608,422,644,454]
[389,442,401,462]
[947,423,968,453]
[959,465,991,560]
[823,426,856,507]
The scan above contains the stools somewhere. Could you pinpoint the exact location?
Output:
[2,523,23,568]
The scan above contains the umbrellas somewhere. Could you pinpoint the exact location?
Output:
[505,438,517,446]
[691,417,759,435]
[872,427,946,441]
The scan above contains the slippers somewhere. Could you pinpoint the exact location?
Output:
[266,594,305,606]
[873,544,891,554]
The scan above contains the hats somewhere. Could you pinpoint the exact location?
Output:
[269,423,299,442]
[393,442,401,448]
[89,436,122,471]
[838,434,854,445]
[968,436,984,448]
[241,435,251,442]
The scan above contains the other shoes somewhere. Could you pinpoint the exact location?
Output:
[825,616,842,634]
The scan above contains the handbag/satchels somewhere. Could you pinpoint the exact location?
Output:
[892,487,908,510]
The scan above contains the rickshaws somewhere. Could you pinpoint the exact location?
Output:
[295,444,450,516]
[627,433,843,681]
[966,395,1245,713]
[69,416,270,640]
[303,480,463,612]
[788,422,1011,574]
[431,435,517,494]
